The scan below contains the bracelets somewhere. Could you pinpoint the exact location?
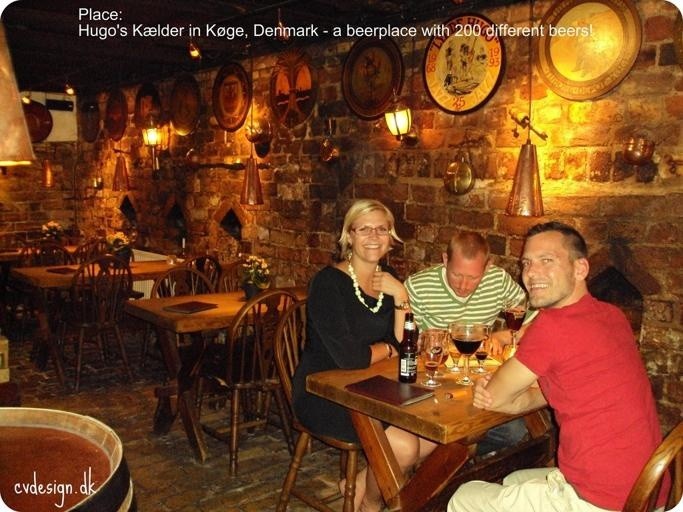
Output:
[385,341,392,358]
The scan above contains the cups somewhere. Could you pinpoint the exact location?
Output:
[166,255,175,265]
[503,343,518,361]
[624,137,656,165]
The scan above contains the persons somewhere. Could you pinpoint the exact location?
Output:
[443,37,488,86]
[402,231,527,336]
[292,198,437,510]
[447,220,671,511]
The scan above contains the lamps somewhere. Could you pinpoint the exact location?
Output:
[187,6,293,59]
[112,135,130,192]
[384,88,419,145]
[500,0,547,218]
[239,47,264,205]
[40,142,55,189]
[139,109,169,176]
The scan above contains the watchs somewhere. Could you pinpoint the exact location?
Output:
[394,296,411,310]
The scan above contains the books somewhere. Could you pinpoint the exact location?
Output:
[0,249,19,253]
[47,266,78,278]
[163,300,218,313]
[344,374,435,408]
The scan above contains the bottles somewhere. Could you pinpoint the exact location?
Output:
[398,313,417,383]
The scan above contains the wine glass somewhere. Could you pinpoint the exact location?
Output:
[419,322,492,388]
[504,309,526,345]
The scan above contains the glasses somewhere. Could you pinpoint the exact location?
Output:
[349,224,391,237]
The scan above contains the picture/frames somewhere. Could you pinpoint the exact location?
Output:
[418,13,505,116]
[22,62,248,146]
[340,34,406,122]
[532,0,643,102]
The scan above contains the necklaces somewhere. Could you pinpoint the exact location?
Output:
[348,252,385,313]
